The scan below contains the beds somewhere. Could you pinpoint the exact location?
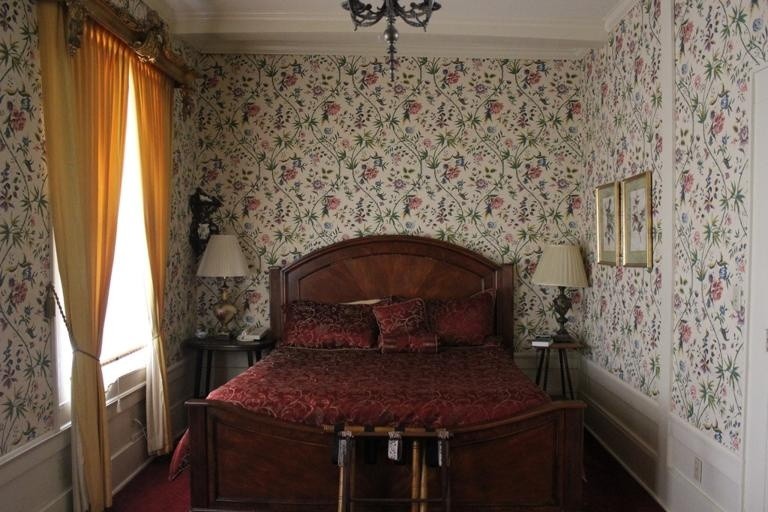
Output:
[183,235,589,511]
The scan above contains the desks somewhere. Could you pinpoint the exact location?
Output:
[181,338,272,399]
[535,342,584,401]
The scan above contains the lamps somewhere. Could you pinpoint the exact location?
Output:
[342,0,443,84]
[530,245,589,343]
[194,234,250,342]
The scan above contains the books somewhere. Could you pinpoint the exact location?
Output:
[531,335,554,349]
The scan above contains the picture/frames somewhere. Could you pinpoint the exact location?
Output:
[595,171,653,273]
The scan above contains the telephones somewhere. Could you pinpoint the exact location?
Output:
[236,324,269,340]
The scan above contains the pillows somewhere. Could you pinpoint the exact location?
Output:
[280,288,505,353]
